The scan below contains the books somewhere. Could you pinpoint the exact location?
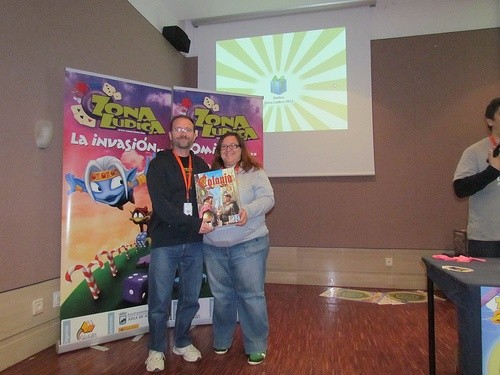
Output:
[193,167,243,224]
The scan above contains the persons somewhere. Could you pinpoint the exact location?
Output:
[452,97,500,258]
[145,115,214,372]
[201,132,275,365]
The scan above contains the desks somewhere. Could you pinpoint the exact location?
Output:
[422,255,500,375]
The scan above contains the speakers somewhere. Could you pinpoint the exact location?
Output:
[162,25,191,53]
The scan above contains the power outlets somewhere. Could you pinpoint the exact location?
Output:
[32,297,44,315]
[385,258,393,267]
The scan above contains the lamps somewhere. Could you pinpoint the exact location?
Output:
[34,119,55,149]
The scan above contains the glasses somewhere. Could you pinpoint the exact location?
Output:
[172,127,194,133]
[220,144,241,149]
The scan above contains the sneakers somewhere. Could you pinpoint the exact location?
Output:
[216,348,227,354]
[145,347,166,372]
[247,352,266,365]
[173,344,202,362]
